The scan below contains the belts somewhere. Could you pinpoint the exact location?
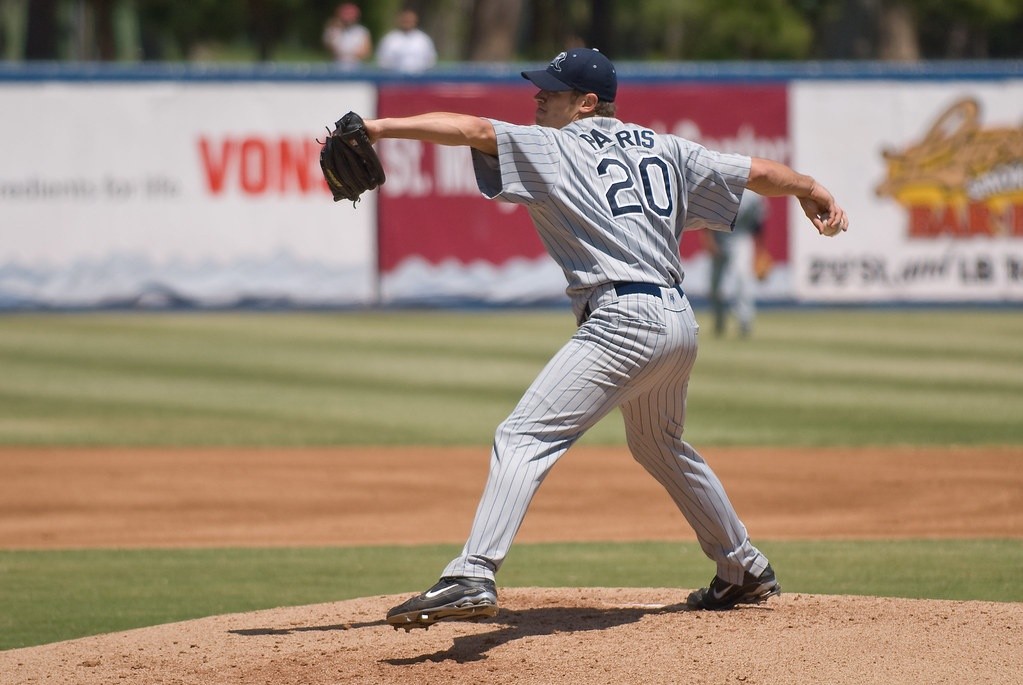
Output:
[584,283,684,317]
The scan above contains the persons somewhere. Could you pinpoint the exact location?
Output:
[709,188,767,340]
[320,1,372,63]
[373,3,438,73]
[321,49,849,633]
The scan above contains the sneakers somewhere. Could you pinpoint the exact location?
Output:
[386,575,500,634]
[686,562,782,612]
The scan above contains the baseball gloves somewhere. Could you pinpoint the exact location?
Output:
[320,110,386,209]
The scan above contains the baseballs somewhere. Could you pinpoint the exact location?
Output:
[819,212,844,237]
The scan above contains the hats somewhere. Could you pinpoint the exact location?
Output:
[520,48,617,102]
